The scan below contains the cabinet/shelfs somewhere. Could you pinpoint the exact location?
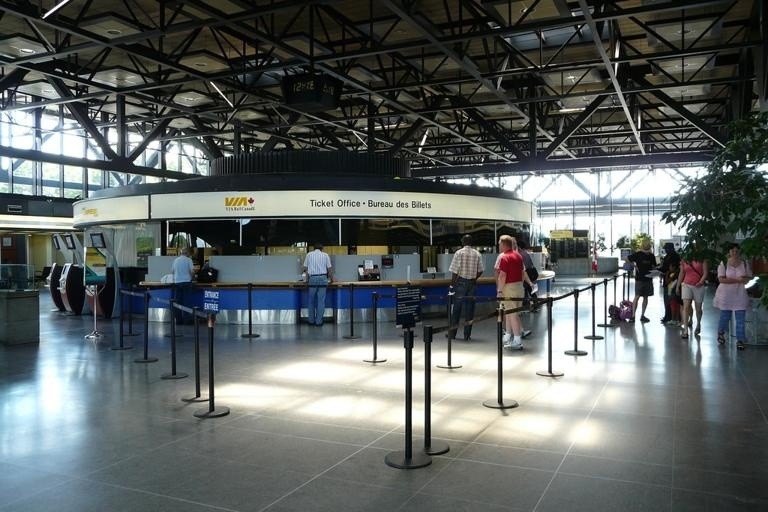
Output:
[0,290,40,346]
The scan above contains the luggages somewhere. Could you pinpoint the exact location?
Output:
[619,273,633,320]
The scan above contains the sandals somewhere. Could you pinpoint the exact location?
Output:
[629,316,744,350]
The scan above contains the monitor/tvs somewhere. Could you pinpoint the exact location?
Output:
[52,236,60,250]
[65,234,76,249]
[90,233,106,248]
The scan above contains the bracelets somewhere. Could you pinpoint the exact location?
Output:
[497,290,503,294]
[448,285,455,289]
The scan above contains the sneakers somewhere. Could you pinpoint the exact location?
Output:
[503,328,531,349]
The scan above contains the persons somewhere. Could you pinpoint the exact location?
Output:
[624,238,708,338]
[303,242,338,326]
[445,235,484,340]
[495,234,524,349]
[517,241,538,312]
[171,247,197,325]
[713,243,752,350]
[494,237,535,337]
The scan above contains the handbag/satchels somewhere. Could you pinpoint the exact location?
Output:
[196,268,218,283]
